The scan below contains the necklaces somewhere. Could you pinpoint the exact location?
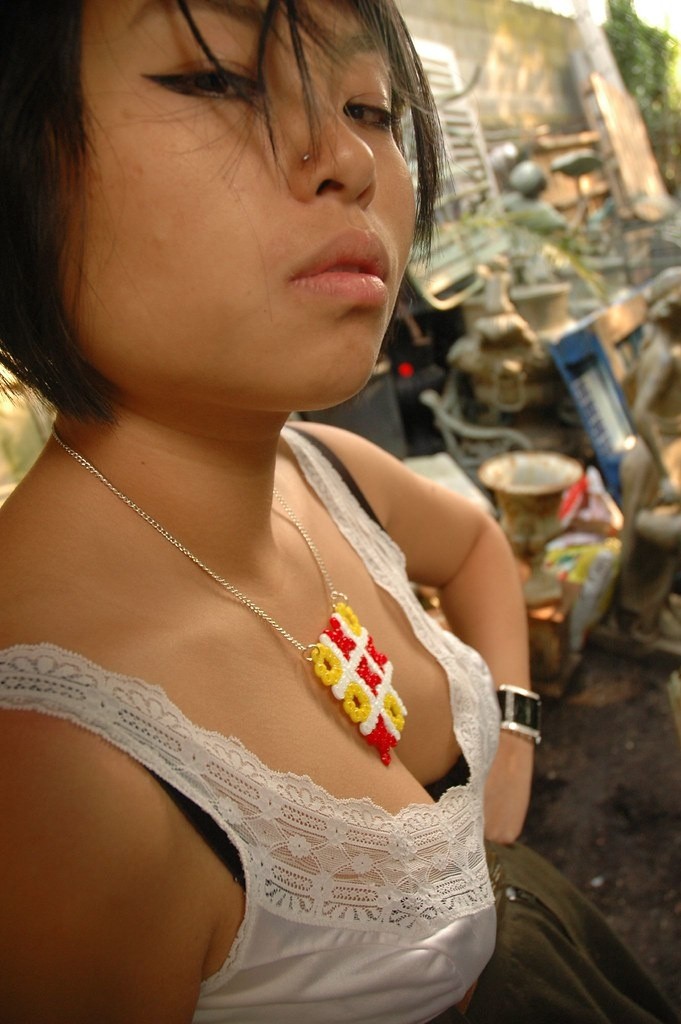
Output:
[47,419,408,766]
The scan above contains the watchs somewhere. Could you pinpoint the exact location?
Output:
[495,683,545,742]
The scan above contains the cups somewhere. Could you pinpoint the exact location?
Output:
[477,451,582,553]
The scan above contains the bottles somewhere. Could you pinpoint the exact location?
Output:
[522,510,623,697]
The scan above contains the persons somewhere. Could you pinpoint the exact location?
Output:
[0,0,681,1024]
